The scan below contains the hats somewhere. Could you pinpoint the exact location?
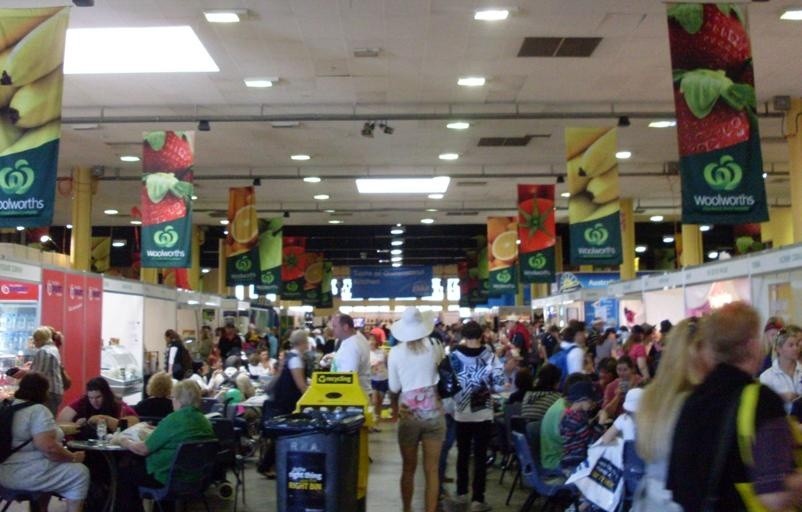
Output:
[622,388,645,412]
[500,315,519,324]
[505,349,525,361]
[388,305,436,341]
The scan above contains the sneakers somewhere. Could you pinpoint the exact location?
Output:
[436,489,492,511]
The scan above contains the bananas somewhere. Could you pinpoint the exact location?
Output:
[567,127,620,224]
[90,236,111,272]
[0,7,70,156]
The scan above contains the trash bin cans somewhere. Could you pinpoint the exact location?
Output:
[292,372,373,512]
[263,410,366,512]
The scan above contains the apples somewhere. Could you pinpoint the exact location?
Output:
[259,229,283,272]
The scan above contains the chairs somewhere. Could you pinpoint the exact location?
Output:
[488,390,581,512]
[199,383,272,510]
[138,438,222,511]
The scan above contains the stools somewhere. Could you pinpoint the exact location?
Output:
[1,486,54,511]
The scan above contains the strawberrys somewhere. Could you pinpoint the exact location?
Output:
[142,130,192,225]
[668,4,755,157]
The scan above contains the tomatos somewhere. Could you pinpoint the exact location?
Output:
[518,185,556,254]
[281,239,305,281]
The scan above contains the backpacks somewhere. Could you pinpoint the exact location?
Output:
[219,371,242,388]
[1,397,39,462]
[547,345,577,387]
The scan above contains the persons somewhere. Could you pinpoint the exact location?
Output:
[0,300,802,512]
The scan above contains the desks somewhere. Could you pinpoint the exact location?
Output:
[66,437,133,512]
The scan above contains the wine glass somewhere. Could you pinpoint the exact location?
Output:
[97,418,108,446]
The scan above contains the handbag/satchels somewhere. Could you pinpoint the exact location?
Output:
[736,417,802,510]
[622,439,648,500]
[436,356,462,400]
